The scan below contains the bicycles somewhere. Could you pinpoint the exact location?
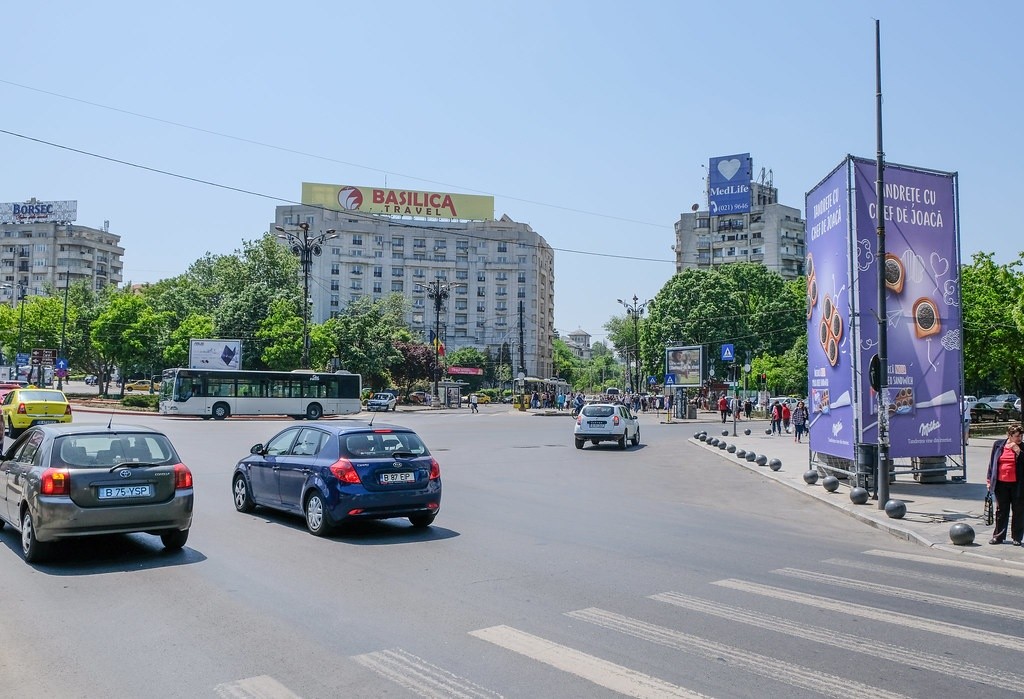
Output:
[572,409,579,420]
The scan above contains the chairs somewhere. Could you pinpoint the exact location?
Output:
[65,445,87,464]
[304,433,328,455]
[110,440,131,451]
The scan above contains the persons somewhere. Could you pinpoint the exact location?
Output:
[792,402,808,444]
[770,400,791,437]
[744,398,752,422]
[447,390,454,408]
[573,394,587,415]
[961,401,972,446]
[697,394,710,410]
[530,390,674,411]
[470,394,479,414]
[730,396,743,420]
[719,394,729,423]
[984,425,1023,546]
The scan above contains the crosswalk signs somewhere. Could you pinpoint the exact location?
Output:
[663,374,676,385]
[721,344,735,362]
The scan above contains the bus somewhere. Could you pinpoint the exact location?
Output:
[605,387,621,402]
[512,377,570,409]
[149,366,362,420]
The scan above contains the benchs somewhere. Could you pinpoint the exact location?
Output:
[348,437,399,451]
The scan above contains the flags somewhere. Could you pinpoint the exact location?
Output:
[430,330,446,357]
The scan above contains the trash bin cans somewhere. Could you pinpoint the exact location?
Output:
[686,404,697,420]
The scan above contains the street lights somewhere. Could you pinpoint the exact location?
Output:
[274,221,339,367]
[415,275,462,407]
[617,295,656,394]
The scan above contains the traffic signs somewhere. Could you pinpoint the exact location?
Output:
[29,356,57,366]
[30,348,58,358]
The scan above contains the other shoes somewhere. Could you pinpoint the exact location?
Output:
[798,440,801,443]
[989,537,1003,544]
[1013,539,1021,545]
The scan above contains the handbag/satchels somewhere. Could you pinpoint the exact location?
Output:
[984,491,993,526]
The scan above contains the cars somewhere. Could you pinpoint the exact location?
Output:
[502,395,516,404]
[125,379,152,392]
[460,392,491,404]
[367,392,397,412]
[724,394,809,417]
[0,423,195,563]
[584,394,600,404]
[0,384,73,438]
[574,403,641,449]
[959,393,1022,422]
[84,371,112,385]
[0,379,30,415]
[409,391,431,403]
[230,420,442,537]
